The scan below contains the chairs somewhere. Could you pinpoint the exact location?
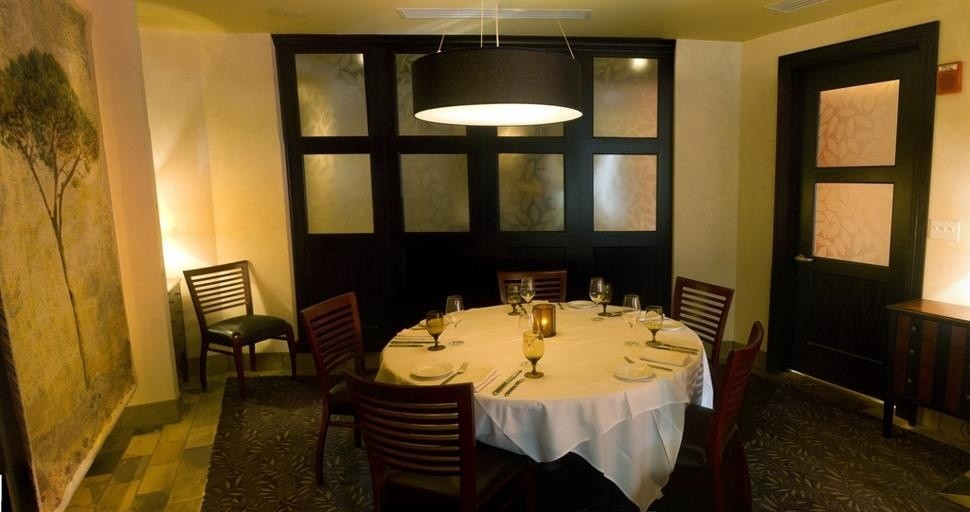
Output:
[297,288,383,488]
[595,319,768,512]
[493,267,570,307]
[180,259,302,400]
[336,364,539,512]
[664,273,737,415]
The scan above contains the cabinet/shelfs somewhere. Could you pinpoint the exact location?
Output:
[876,294,969,442]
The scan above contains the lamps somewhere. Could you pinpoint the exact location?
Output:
[404,0,588,132]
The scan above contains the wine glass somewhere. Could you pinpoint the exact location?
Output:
[507,275,535,321]
[588,276,663,345]
[523,330,545,380]
[425,292,465,351]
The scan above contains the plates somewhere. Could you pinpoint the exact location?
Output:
[660,322,684,330]
[614,363,656,381]
[411,361,454,378]
[418,316,449,326]
[568,300,596,308]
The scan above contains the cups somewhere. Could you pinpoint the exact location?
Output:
[531,303,557,338]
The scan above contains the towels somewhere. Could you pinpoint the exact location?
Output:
[638,344,691,371]
[460,367,500,393]
[626,310,664,323]
[390,325,445,344]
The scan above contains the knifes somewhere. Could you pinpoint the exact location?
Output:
[494,368,525,398]
[649,341,701,356]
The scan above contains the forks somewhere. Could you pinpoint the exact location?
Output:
[442,361,468,385]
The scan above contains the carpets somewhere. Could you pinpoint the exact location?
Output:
[195,360,969,511]
